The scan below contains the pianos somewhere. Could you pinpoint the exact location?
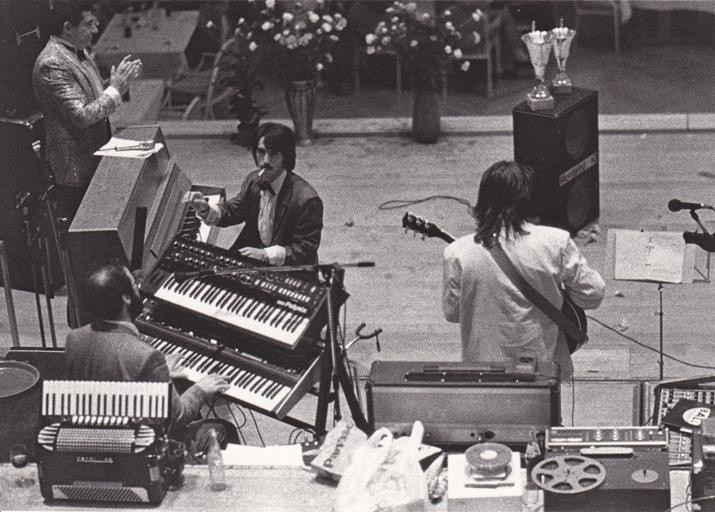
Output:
[142,232,349,369]
[40,378,172,424]
[130,300,349,441]
[67,125,225,289]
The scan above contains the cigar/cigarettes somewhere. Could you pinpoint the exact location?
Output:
[258,168,266,176]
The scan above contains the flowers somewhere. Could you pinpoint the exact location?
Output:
[247,0,347,81]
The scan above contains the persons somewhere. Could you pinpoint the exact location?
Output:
[33,0,143,224]
[63,262,241,465]
[181,122,323,280]
[443,160,606,426]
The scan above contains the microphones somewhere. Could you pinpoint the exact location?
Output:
[667,198,712,213]
[173,269,217,283]
[115,139,155,151]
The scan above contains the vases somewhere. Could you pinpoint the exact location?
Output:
[286,80,316,146]
[412,89,440,144]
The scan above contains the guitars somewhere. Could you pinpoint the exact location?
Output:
[402,212,587,354]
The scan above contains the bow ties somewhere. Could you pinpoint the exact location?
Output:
[74,48,87,62]
[258,180,276,198]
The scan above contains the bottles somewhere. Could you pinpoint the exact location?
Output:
[123,12,133,40]
[524,427,542,487]
[203,429,226,490]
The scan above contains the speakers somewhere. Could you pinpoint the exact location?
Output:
[512,84,600,239]
[364,357,563,454]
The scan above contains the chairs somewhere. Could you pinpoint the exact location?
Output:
[161,36,241,120]
[352,0,620,98]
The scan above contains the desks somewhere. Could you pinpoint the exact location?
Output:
[91,9,200,83]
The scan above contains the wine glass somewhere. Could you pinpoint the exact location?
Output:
[521,30,553,102]
[551,28,574,90]
[9,444,32,490]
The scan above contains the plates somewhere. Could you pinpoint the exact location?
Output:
[0,358,41,399]
